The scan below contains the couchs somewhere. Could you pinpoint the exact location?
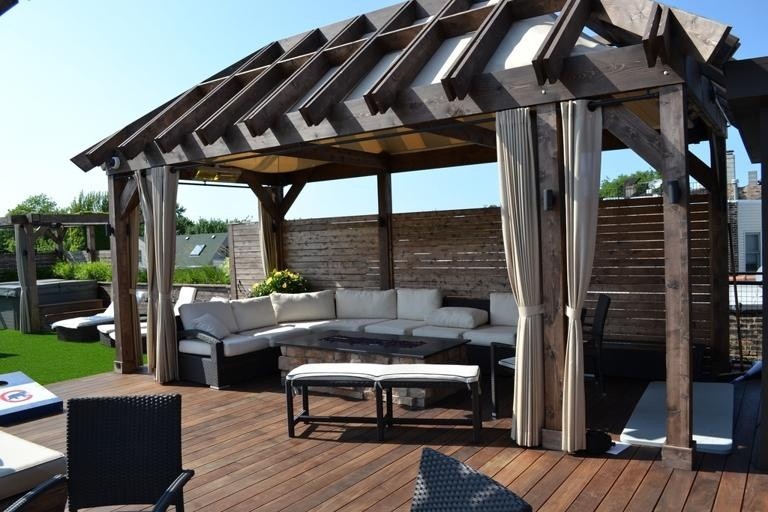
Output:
[173,285,523,390]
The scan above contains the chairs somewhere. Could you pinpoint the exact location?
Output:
[51,284,198,348]
[2,393,197,512]
[488,295,613,418]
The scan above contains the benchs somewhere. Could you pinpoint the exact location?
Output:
[284,362,485,446]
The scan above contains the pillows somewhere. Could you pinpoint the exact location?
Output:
[192,313,232,340]
